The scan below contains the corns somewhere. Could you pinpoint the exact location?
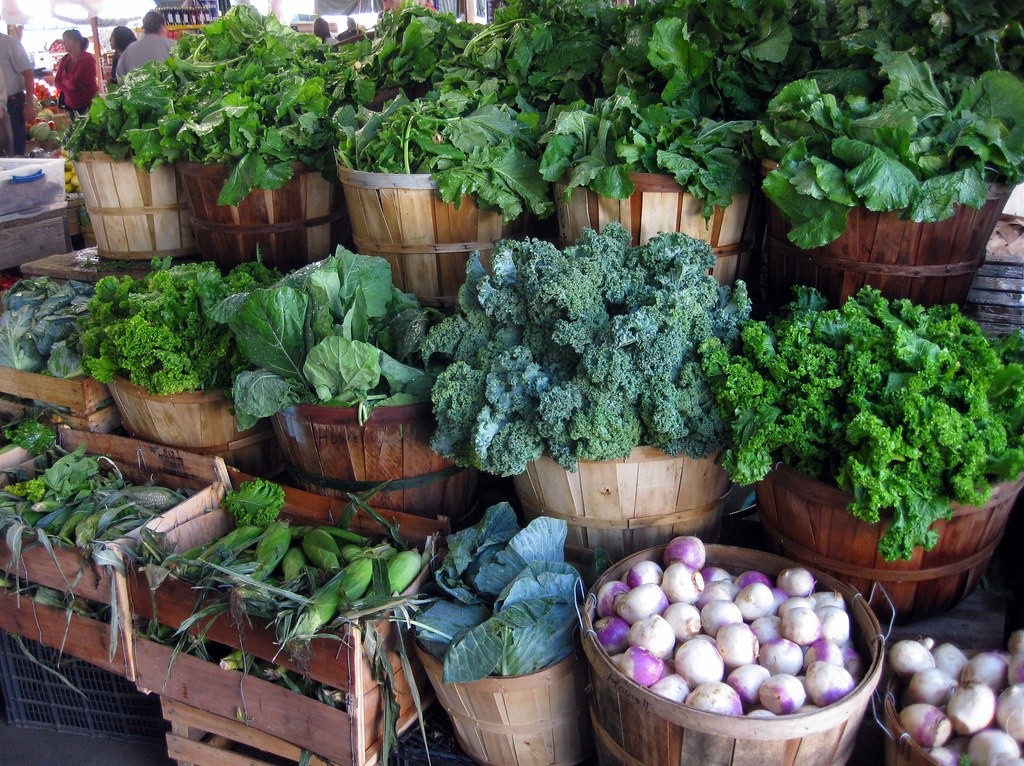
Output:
[1,484,186,549]
[147,523,424,708]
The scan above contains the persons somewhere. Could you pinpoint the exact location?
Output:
[313,17,334,44]
[109,26,138,84]
[116,10,179,86]
[337,18,362,41]
[0,23,34,158]
[54,29,98,123]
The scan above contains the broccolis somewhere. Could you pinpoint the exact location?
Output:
[423,224,753,477]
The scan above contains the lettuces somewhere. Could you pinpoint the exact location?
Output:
[79,256,282,395]
[220,479,285,526]
[702,285,1024,560]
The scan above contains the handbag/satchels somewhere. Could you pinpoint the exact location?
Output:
[59,91,70,109]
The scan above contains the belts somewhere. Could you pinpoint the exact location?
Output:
[8,92,26,101]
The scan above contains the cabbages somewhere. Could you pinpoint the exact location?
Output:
[0,277,93,379]
[414,499,583,687]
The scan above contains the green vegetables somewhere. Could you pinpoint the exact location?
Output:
[43,446,102,502]
[213,246,444,430]
[68,0,1024,245]
